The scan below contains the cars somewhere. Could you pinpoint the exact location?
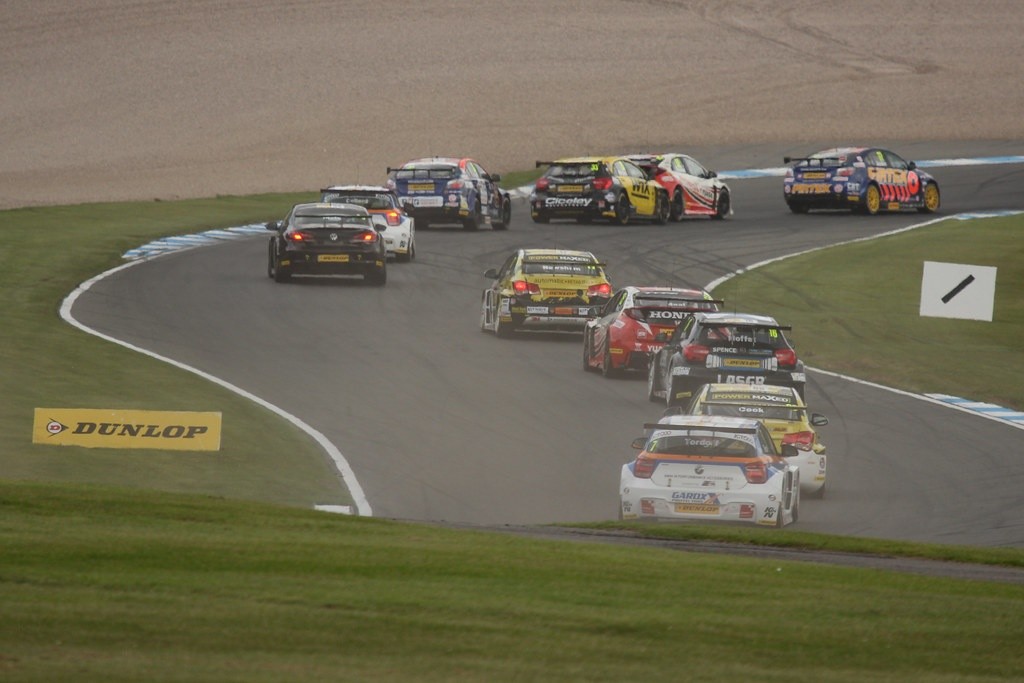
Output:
[666,382,826,496]
[321,184,416,261]
[530,156,671,226]
[618,415,800,529]
[386,156,511,232]
[581,285,721,375]
[481,249,617,338]
[649,309,804,419]
[782,147,941,216]
[620,154,732,222]
[265,203,388,282]
